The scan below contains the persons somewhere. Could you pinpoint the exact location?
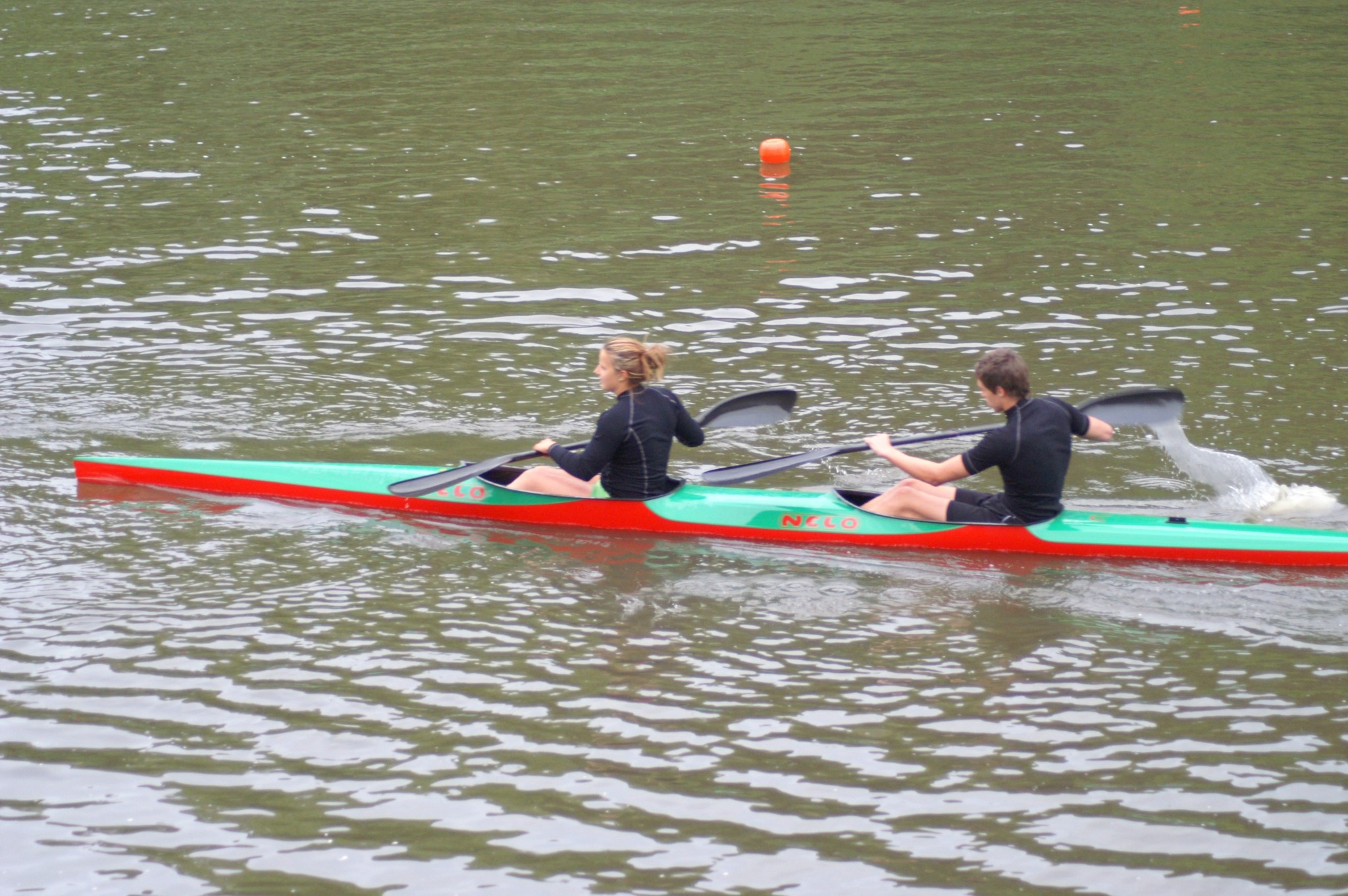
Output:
[506,339,704,499]
[857,348,1113,524]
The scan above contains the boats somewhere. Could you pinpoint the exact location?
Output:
[73,449,1348,569]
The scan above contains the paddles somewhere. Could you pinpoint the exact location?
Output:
[699,381,1188,489]
[387,386,800,498]
